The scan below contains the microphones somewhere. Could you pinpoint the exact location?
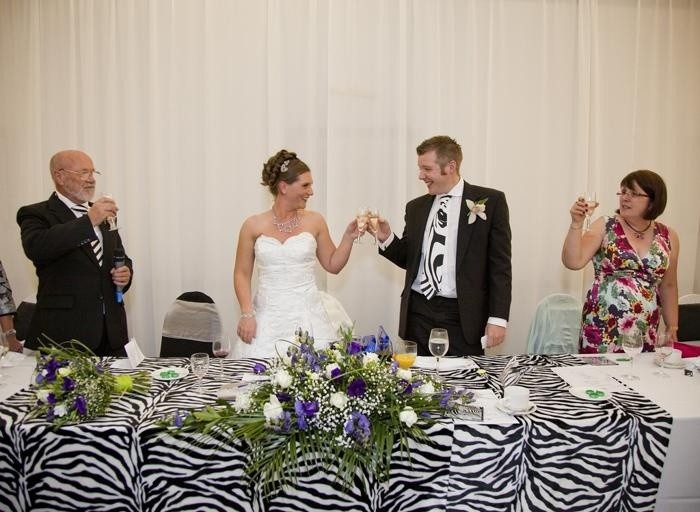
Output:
[113,245,125,303]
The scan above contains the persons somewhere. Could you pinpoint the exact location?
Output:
[368,135,513,357]
[562,168,681,353]
[0,260,25,355]
[14,149,133,358]
[223,149,369,359]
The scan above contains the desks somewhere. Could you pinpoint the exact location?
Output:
[0,342,700,510]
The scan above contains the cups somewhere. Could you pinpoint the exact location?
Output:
[661,347,682,364]
[504,386,529,411]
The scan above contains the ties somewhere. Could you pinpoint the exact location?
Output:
[72,204,103,268]
[421,195,452,301]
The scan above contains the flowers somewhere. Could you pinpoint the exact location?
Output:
[23,327,151,432]
[158,322,486,501]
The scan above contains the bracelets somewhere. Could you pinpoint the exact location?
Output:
[664,326,680,331]
[5,328,17,337]
[238,311,256,319]
[568,223,583,230]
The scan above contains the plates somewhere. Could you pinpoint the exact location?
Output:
[495,399,537,415]
[151,367,190,380]
[568,387,612,401]
[656,360,691,369]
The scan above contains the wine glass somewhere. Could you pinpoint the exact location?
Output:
[191,353,209,393]
[652,333,674,378]
[428,328,449,381]
[352,206,383,245]
[582,191,596,232]
[621,329,643,381]
[212,335,230,379]
[0,333,11,388]
[100,191,120,232]
[392,340,418,370]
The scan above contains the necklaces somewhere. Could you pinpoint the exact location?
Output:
[271,208,301,232]
[623,218,651,239]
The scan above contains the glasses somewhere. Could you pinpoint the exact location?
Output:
[57,168,101,180]
[616,191,649,199]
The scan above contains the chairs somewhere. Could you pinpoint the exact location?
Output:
[159,289,223,358]
[678,293,699,344]
[11,293,40,356]
[526,293,585,354]
